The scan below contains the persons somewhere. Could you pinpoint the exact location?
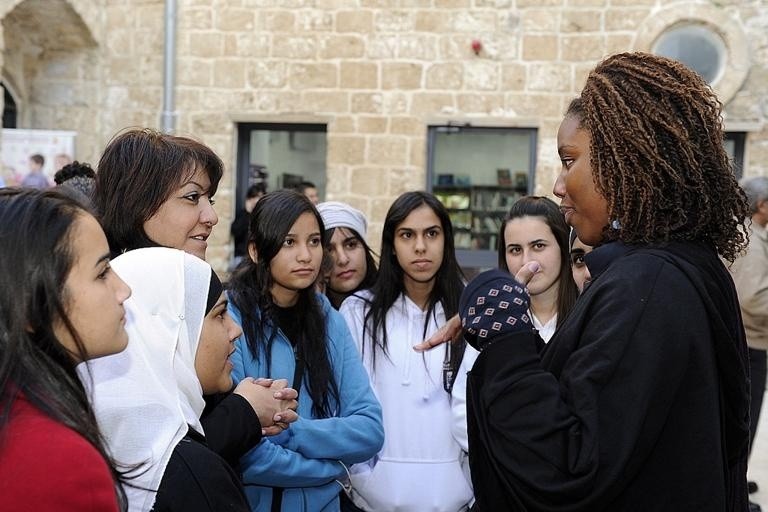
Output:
[74,245,254,508]
[20,154,52,193]
[309,198,376,317]
[0,183,134,512]
[87,127,299,458]
[448,194,591,499]
[568,217,593,295]
[413,51,757,510]
[294,180,318,208]
[231,182,266,258]
[340,192,490,510]
[48,160,101,188]
[217,190,388,511]
[722,175,766,508]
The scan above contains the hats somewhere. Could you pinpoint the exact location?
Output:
[316,201,368,239]
